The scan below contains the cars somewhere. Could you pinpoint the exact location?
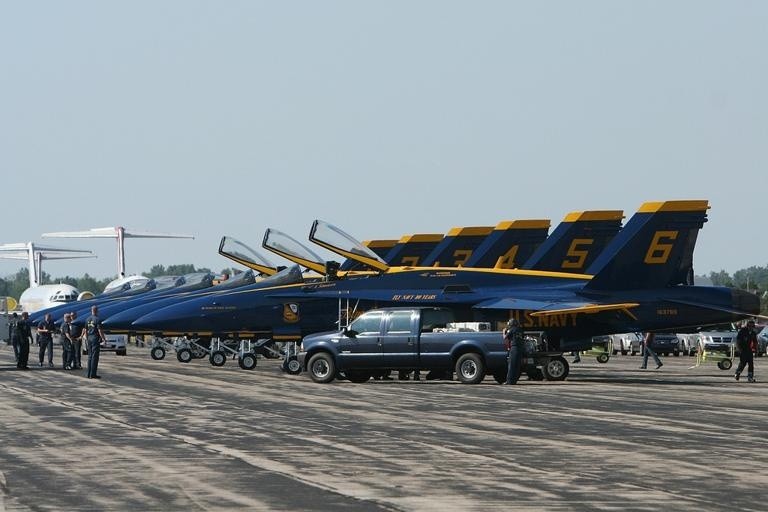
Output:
[595,322,768,361]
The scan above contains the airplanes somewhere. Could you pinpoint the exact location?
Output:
[0,195,760,376]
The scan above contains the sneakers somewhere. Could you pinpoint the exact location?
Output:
[90,375,100,378]
[735,372,740,380]
[48,361,54,367]
[62,365,83,370]
[747,376,755,382]
[38,361,42,366]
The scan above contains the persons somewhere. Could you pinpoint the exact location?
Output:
[637,331,663,371]
[6,304,107,380]
[732,320,760,383]
[501,318,525,385]
[572,351,581,363]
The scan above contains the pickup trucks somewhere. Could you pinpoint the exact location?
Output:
[294,304,538,385]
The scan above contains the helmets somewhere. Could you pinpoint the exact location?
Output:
[507,318,518,325]
